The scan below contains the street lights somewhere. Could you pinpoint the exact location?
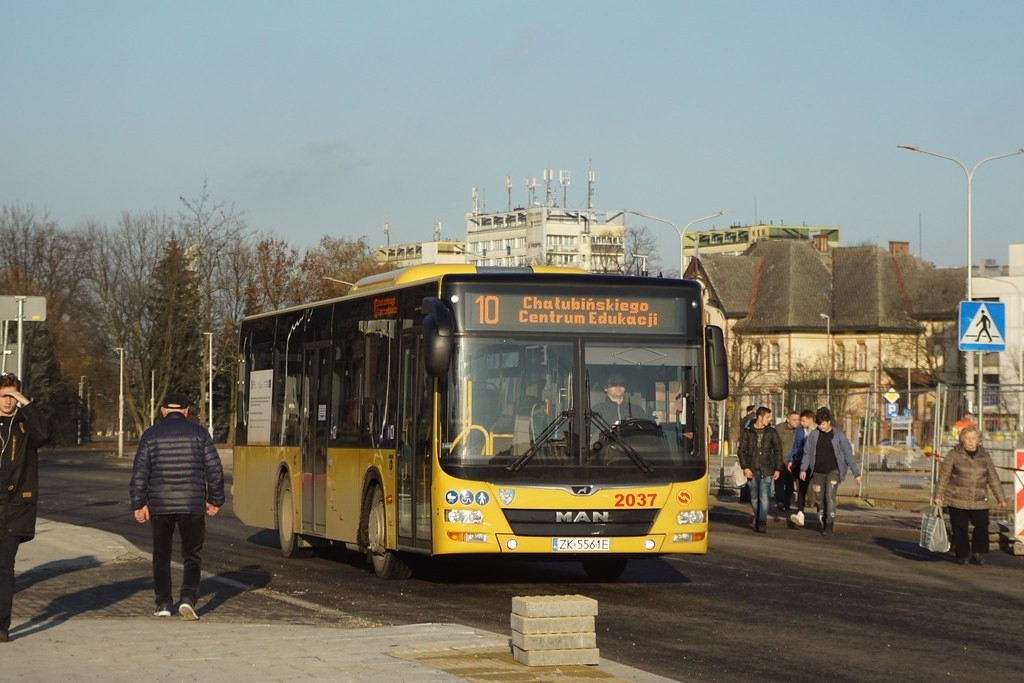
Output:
[114,347,125,457]
[203,331,216,439]
[150,367,157,426]
[895,143,1024,412]
[623,210,730,279]
[819,312,832,406]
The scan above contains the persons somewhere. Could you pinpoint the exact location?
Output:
[787,410,817,527]
[738,405,782,532]
[955,412,974,432]
[0,373,57,643]
[934,427,1007,565]
[131,393,225,620]
[591,374,645,435]
[799,407,861,536]
[774,411,799,510]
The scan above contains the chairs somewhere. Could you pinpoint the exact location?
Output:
[336,383,561,448]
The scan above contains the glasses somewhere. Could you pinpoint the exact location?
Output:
[606,381,626,387]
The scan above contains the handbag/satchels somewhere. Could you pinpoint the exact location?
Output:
[732,461,747,489]
[919,504,948,553]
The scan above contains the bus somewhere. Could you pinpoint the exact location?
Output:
[230,261,729,581]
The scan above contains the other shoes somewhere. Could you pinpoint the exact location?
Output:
[957,556,967,565]
[0,630,9,642]
[822,529,833,535]
[755,520,767,533]
[785,505,790,511]
[154,596,173,616]
[790,511,805,526]
[776,502,787,513]
[179,596,199,621]
[817,510,824,529]
[740,495,751,503]
[972,552,986,565]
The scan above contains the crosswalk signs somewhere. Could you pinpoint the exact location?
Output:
[958,300,1006,353]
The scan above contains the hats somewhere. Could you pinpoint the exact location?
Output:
[747,405,755,412]
[162,393,190,409]
